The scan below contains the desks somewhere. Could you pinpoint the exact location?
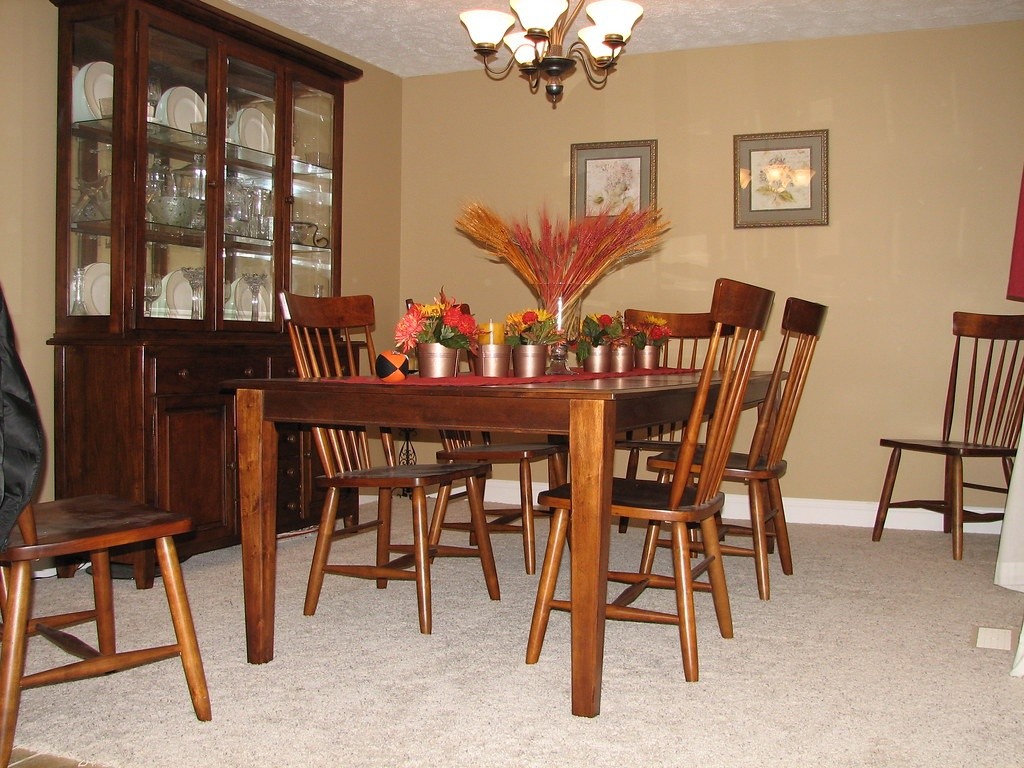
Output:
[221,372,789,718]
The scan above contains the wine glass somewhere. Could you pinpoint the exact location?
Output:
[223,280,231,320]
[144,273,162,317]
[226,99,237,144]
[226,177,255,222]
[148,77,163,124]
[291,120,301,160]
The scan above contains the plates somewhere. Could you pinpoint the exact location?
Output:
[235,275,272,321]
[166,269,203,319]
[84,264,110,315]
[84,61,114,119]
[239,108,275,153]
[167,86,207,134]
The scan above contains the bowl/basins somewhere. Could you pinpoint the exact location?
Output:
[147,197,200,228]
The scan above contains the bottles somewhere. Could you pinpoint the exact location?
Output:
[405,299,419,374]
[151,151,174,196]
[313,284,323,297]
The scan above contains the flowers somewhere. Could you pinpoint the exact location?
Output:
[393,290,492,357]
[505,309,562,346]
[575,310,672,360]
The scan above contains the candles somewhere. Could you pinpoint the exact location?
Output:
[478,318,504,346]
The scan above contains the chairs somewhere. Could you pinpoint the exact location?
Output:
[648,296,829,598]
[0,287,212,768]
[405,299,569,574]
[872,312,1024,560]
[280,279,491,681]
[526,277,775,683]
[620,309,735,557]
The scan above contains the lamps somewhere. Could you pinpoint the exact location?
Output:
[460,0,644,106]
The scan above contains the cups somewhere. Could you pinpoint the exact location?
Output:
[290,225,309,243]
[190,122,207,136]
[224,218,246,235]
[99,97,114,119]
[305,151,320,165]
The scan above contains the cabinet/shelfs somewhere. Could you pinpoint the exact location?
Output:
[49,0,351,589]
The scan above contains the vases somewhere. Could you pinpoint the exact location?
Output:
[635,345,660,370]
[530,283,591,343]
[610,344,633,373]
[513,345,546,378]
[418,343,457,378]
[583,345,610,373]
[473,343,511,378]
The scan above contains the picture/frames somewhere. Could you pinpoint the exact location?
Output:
[570,139,658,228]
[734,129,829,228]
